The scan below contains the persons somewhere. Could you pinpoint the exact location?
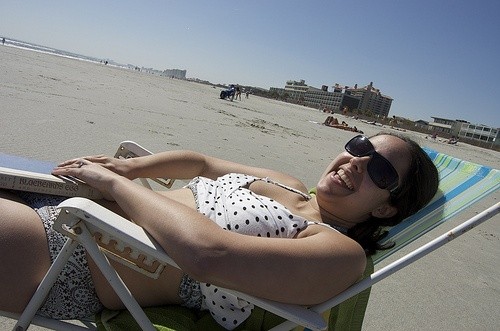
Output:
[0,132,439,331]
[220,84,249,101]
[324,116,358,131]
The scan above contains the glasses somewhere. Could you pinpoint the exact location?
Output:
[345,135,399,193]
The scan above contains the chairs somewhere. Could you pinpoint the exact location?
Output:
[0,141,500,331]
